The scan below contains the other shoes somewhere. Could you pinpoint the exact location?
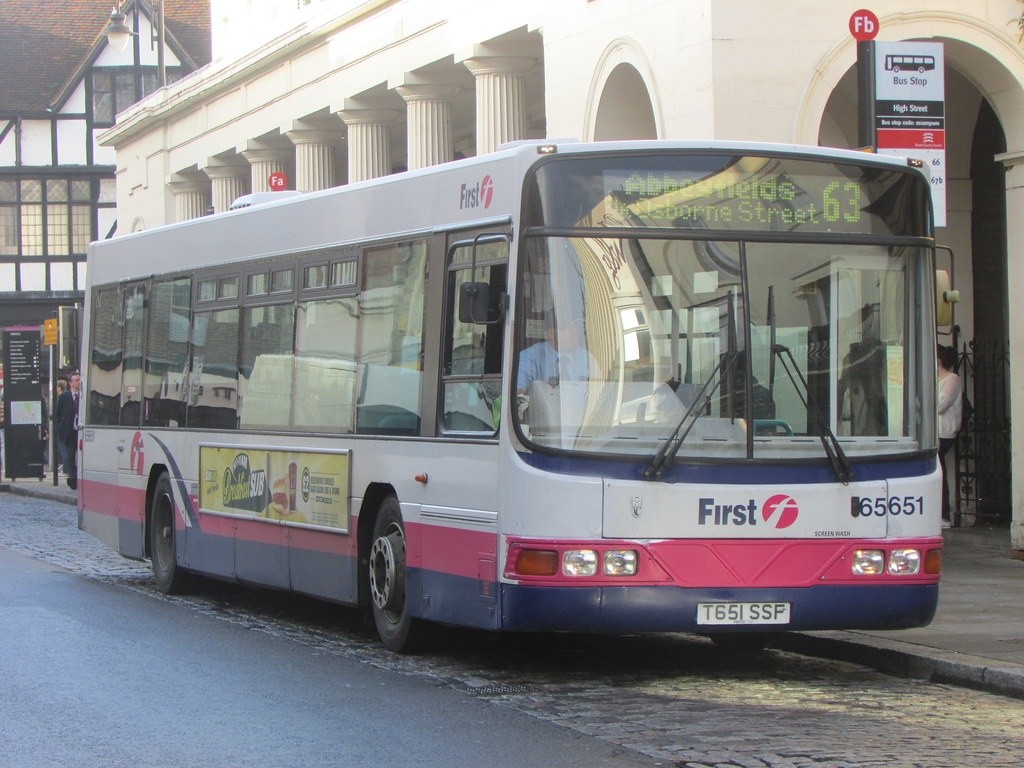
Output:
[67,478,77,490]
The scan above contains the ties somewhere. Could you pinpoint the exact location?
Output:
[72,393,79,415]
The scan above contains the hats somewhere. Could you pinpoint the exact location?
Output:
[547,307,584,331]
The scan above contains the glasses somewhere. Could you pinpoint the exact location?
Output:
[70,379,81,382]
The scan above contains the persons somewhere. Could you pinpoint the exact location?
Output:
[517,306,604,399]
[935,342,963,530]
[57,373,80,491]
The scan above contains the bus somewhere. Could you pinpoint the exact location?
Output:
[75,136,959,654]
